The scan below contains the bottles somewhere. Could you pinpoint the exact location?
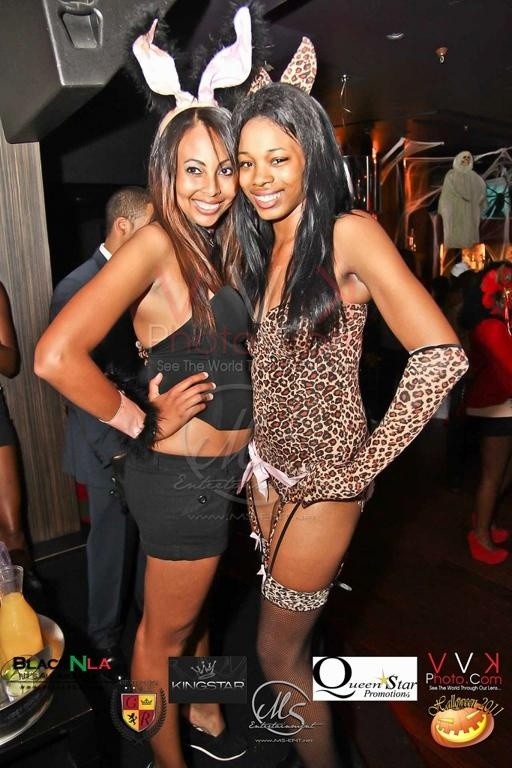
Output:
[0,563,46,665]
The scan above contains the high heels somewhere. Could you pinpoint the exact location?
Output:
[467,511,509,565]
[179,719,246,760]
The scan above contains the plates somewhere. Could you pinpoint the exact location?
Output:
[1,606,68,735]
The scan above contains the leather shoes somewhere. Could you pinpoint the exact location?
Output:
[97,649,127,682]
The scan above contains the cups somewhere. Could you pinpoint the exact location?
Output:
[1,655,45,702]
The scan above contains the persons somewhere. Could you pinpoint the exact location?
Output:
[0,280,31,606]
